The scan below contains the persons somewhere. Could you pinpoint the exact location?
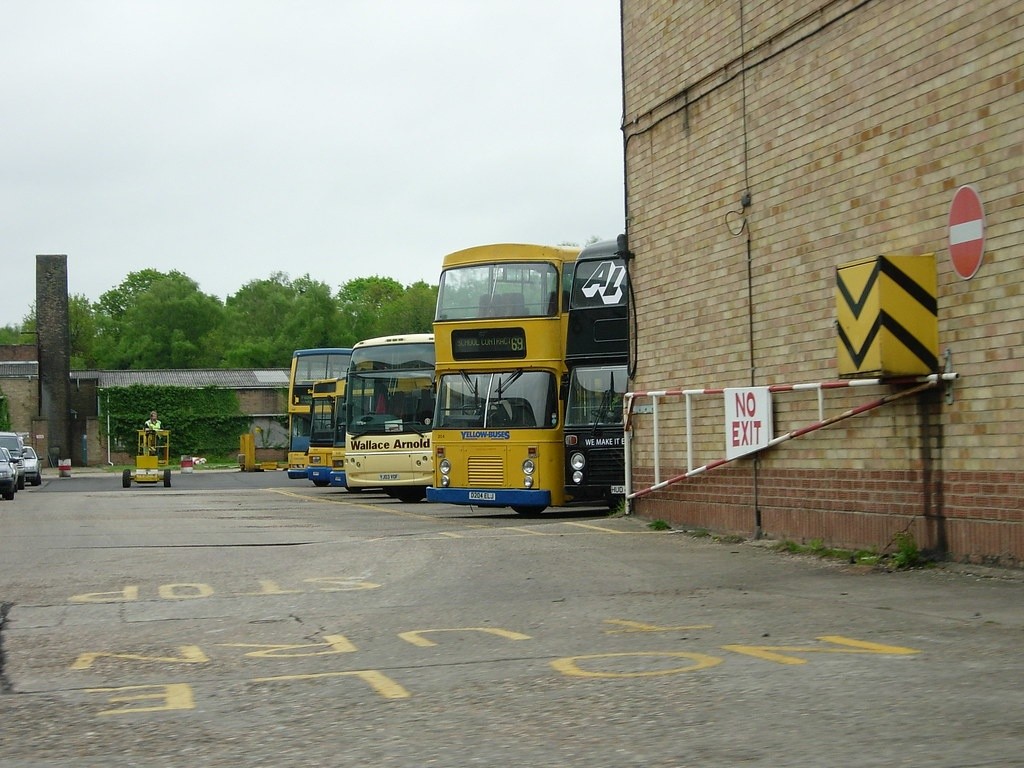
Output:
[144,411,165,456]
[606,390,620,411]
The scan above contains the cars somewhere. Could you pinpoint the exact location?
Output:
[0,447,19,500]
[0,432,25,490]
[23,446,42,486]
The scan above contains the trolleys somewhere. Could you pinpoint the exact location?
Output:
[123,429,171,488]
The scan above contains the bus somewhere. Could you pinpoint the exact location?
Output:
[425,244,585,515]
[345,333,435,503]
[330,375,434,495]
[561,240,629,508]
[307,377,339,481]
[287,348,354,486]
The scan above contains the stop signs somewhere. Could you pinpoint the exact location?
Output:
[946,185,986,281]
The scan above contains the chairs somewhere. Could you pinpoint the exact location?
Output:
[385,389,435,427]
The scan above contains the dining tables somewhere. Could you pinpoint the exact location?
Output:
[479,290,570,318]
